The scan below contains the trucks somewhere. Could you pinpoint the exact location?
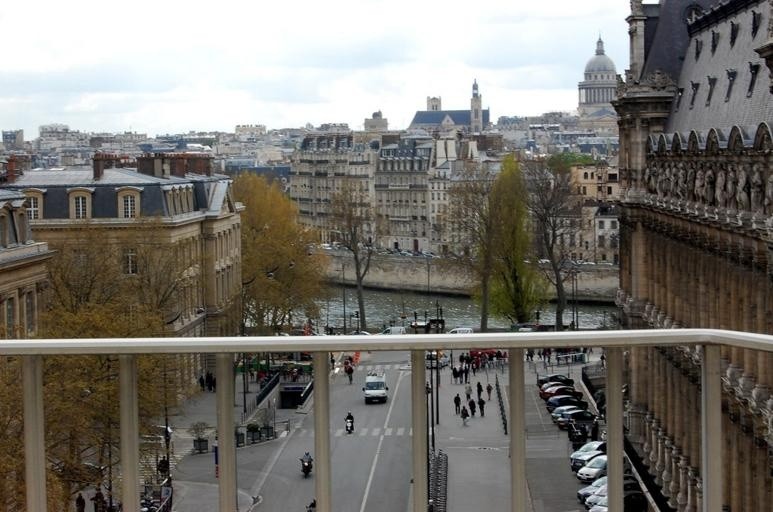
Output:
[382,326,406,334]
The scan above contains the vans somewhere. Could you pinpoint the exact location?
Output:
[449,328,473,334]
[362,371,389,404]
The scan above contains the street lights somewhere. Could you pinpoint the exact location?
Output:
[158,306,205,477]
[241,272,276,414]
[425,380,432,505]
[563,269,582,330]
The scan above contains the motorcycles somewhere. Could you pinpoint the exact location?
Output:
[344,417,354,434]
[299,458,313,478]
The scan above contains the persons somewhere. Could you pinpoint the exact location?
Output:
[330,355,354,381]
[345,411,354,431]
[306,498,316,509]
[250,364,303,384]
[355,330,359,335]
[75,488,117,511]
[526,347,550,363]
[329,327,334,335]
[300,451,314,473]
[199,371,217,393]
[452,351,510,421]
[158,457,167,479]
[645,161,773,216]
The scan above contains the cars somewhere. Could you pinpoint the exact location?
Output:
[425,352,450,369]
[140,425,175,450]
[537,374,648,512]
